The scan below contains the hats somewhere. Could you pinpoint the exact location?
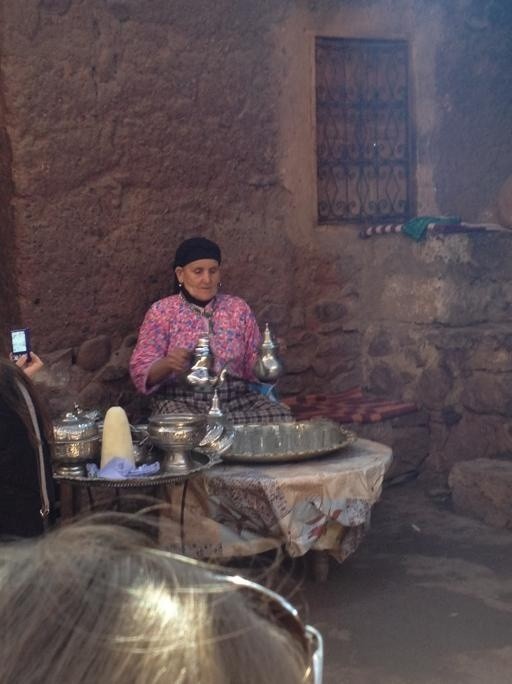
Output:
[174,237,221,268]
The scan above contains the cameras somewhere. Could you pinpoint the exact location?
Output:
[10,329,32,363]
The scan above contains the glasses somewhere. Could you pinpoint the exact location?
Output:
[141,548,325,684]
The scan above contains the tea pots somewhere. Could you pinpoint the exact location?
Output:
[253,322,282,384]
[184,336,228,393]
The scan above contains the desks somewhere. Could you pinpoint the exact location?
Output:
[42,432,212,558]
[164,434,400,583]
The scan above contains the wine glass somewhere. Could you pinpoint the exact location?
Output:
[148,413,208,474]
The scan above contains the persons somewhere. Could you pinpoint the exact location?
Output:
[5,349,44,379]
[131,235,295,427]
[2,495,326,683]
